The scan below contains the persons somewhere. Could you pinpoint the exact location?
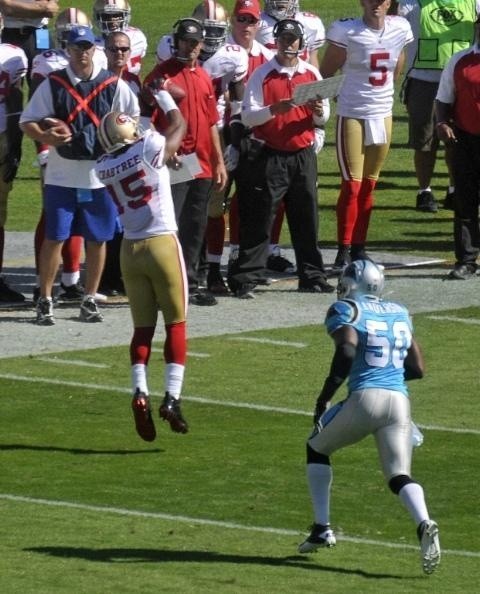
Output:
[400,0,480,280]
[298,258,449,576]
[144,0,335,304]
[321,1,414,269]
[0,0,146,324]
[94,77,192,441]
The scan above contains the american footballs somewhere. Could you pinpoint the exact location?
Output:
[144,76,187,103]
[37,116,73,144]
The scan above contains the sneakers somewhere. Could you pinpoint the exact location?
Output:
[416,191,438,212]
[417,519,441,575]
[132,386,156,441]
[160,392,189,433]
[443,191,455,210]
[448,263,478,279]
[297,524,336,553]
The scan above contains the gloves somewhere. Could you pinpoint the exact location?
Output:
[0,153,22,183]
[224,144,240,171]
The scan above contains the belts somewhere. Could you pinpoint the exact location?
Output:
[2,24,48,36]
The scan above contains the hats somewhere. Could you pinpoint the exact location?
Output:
[177,19,203,41]
[234,0,261,20]
[276,20,302,38]
[68,25,96,46]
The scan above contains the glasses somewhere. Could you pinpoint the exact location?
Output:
[235,14,258,24]
[106,45,130,52]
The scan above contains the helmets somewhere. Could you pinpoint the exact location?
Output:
[56,6,93,43]
[97,110,147,153]
[338,259,385,301]
[192,0,230,52]
[264,0,300,20]
[93,0,131,34]
